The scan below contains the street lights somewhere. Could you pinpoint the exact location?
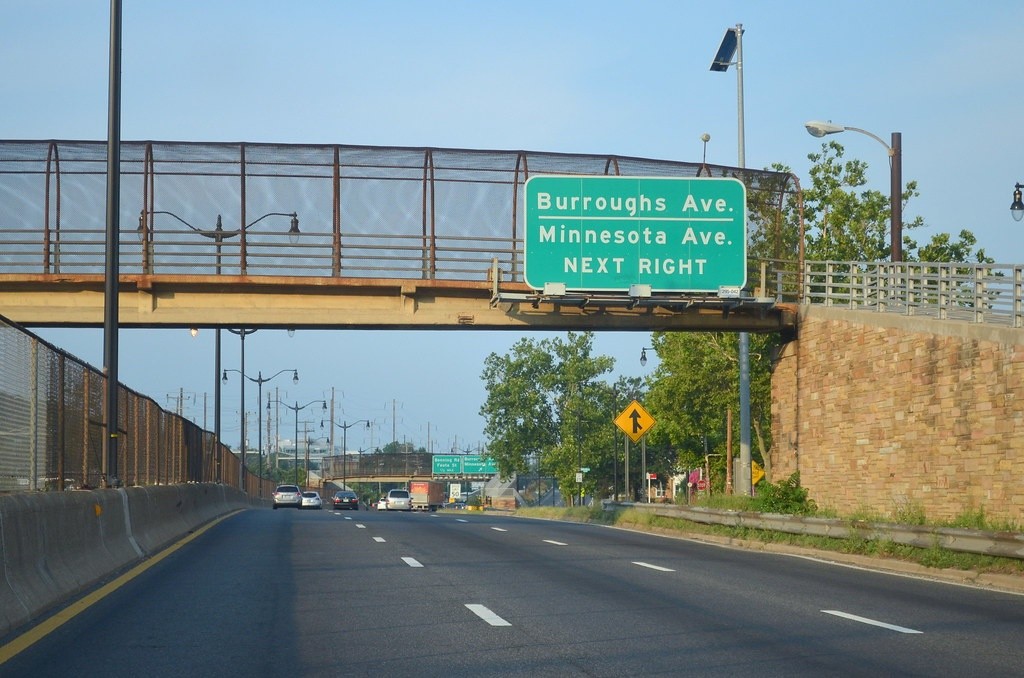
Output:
[806,118,904,263]
[136,203,303,480]
[708,22,746,184]
[220,365,373,491]
[450,445,482,500]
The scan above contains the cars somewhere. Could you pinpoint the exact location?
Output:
[377,498,387,511]
[331,491,359,510]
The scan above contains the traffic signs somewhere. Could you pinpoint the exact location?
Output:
[463,455,500,473]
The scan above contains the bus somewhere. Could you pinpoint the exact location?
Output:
[461,491,472,502]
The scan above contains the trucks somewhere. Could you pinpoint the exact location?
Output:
[410,481,446,512]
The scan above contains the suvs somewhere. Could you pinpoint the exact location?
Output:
[302,491,322,508]
[271,484,303,510]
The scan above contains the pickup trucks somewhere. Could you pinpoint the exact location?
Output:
[386,489,413,511]
